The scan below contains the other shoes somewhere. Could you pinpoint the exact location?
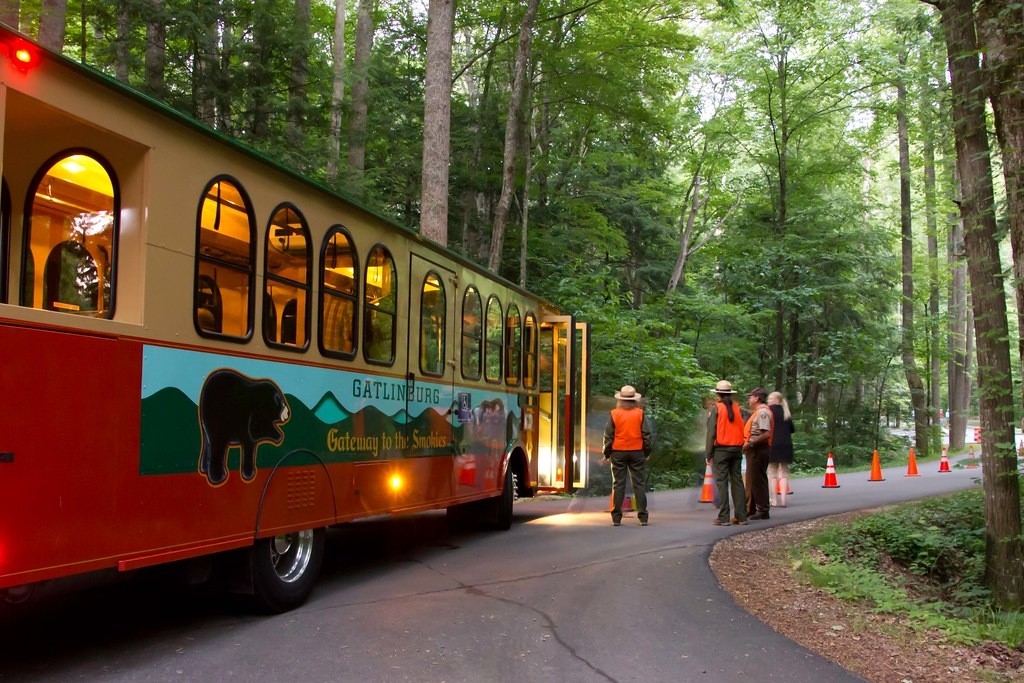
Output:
[732,518,748,525]
[749,511,770,520]
[612,523,620,526]
[637,520,648,525]
[712,518,730,526]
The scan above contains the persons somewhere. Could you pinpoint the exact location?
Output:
[706,380,749,525]
[767,392,795,507]
[325,285,352,351]
[598,386,653,526]
[743,388,774,520]
[940,409,944,425]
[946,409,949,422]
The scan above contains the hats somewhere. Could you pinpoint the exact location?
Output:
[614,385,641,401]
[710,380,737,393]
[748,387,768,396]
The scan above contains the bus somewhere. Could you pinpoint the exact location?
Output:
[0,23,594,615]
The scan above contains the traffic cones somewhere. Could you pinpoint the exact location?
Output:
[965,445,978,469]
[867,450,886,481]
[603,484,633,513]
[1018,439,1024,457]
[937,445,953,472]
[696,462,717,503]
[821,452,841,488]
[775,465,794,495]
[903,447,923,477]
